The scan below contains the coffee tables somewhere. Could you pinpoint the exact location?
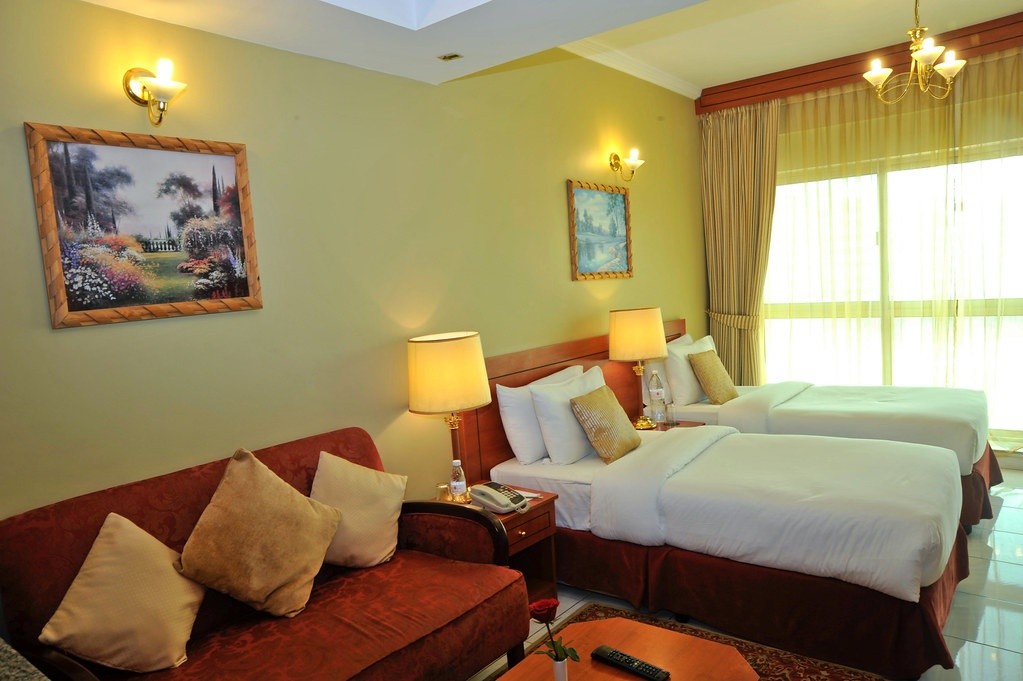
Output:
[495,616,760,681]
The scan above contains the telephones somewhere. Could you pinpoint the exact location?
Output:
[469,481,527,514]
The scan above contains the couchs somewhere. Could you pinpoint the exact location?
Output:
[0,431,530,681]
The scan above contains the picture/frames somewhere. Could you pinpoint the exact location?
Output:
[24,121,263,330]
[567,180,634,281]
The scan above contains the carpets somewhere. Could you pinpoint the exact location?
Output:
[485,601,888,681]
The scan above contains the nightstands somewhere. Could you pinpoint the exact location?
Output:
[464,481,559,617]
[651,420,706,429]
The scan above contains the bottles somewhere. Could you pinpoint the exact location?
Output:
[450,459,468,504]
[647,370,668,422]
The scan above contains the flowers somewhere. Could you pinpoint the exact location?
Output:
[529,598,581,662]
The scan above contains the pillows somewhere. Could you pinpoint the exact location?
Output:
[641,333,693,404]
[172,447,342,618]
[688,350,739,404]
[664,335,718,406]
[570,385,641,464]
[309,450,407,569]
[37,511,207,673]
[495,365,583,464]
[529,365,605,464]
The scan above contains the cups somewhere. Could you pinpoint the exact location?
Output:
[664,404,676,426]
[436,481,451,502]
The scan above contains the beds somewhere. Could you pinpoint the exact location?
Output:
[450,337,968,681]
[609,319,1004,534]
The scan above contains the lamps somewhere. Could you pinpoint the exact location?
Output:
[862,0,967,105]
[610,308,668,430]
[610,148,645,181]
[407,331,492,503]
[123,59,187,127]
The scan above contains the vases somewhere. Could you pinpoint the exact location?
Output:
[554,658,568,681]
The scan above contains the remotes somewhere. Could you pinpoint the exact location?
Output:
[591,645,670,681]
